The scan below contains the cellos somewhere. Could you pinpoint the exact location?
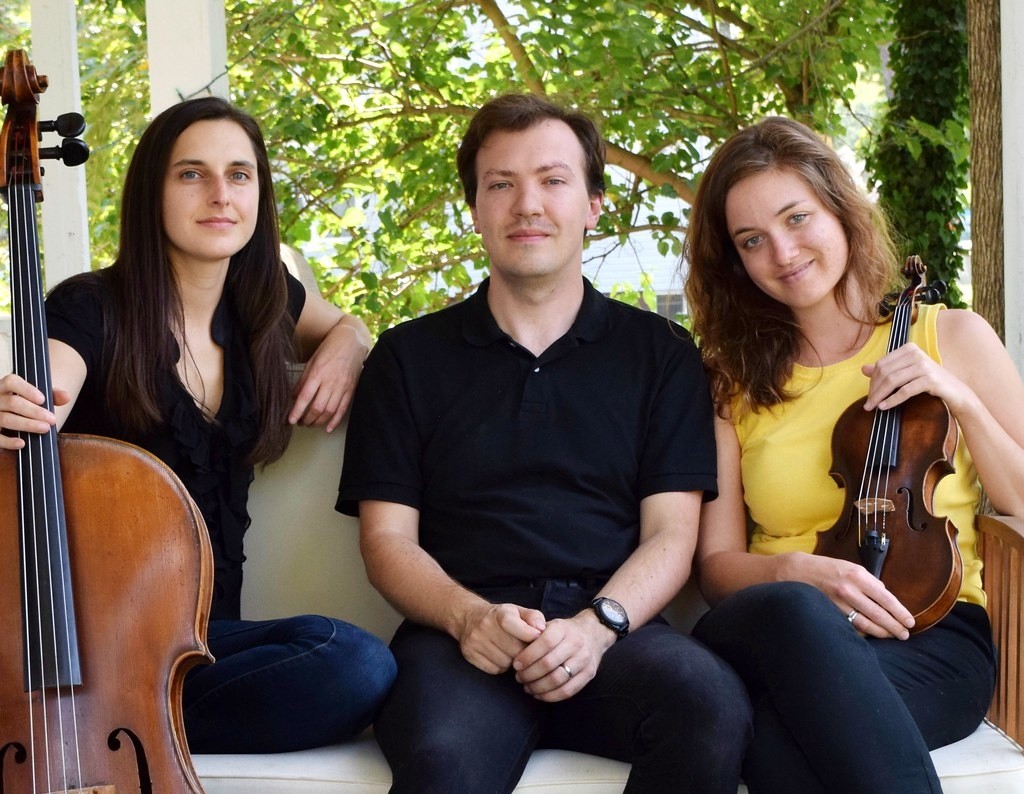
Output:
[1,41,222,792]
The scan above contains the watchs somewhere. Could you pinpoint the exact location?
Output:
[584,597,629,640]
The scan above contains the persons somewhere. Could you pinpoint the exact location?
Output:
[332,91,759,794]
[1,98,396,754]
[680,117,1024,794]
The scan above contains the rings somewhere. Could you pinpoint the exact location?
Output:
[559,664,573,677]
[847,610,858,622]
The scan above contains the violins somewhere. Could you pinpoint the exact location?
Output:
[810,253,964,637]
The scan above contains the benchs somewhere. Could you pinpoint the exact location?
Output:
[189,362,1024,794]
